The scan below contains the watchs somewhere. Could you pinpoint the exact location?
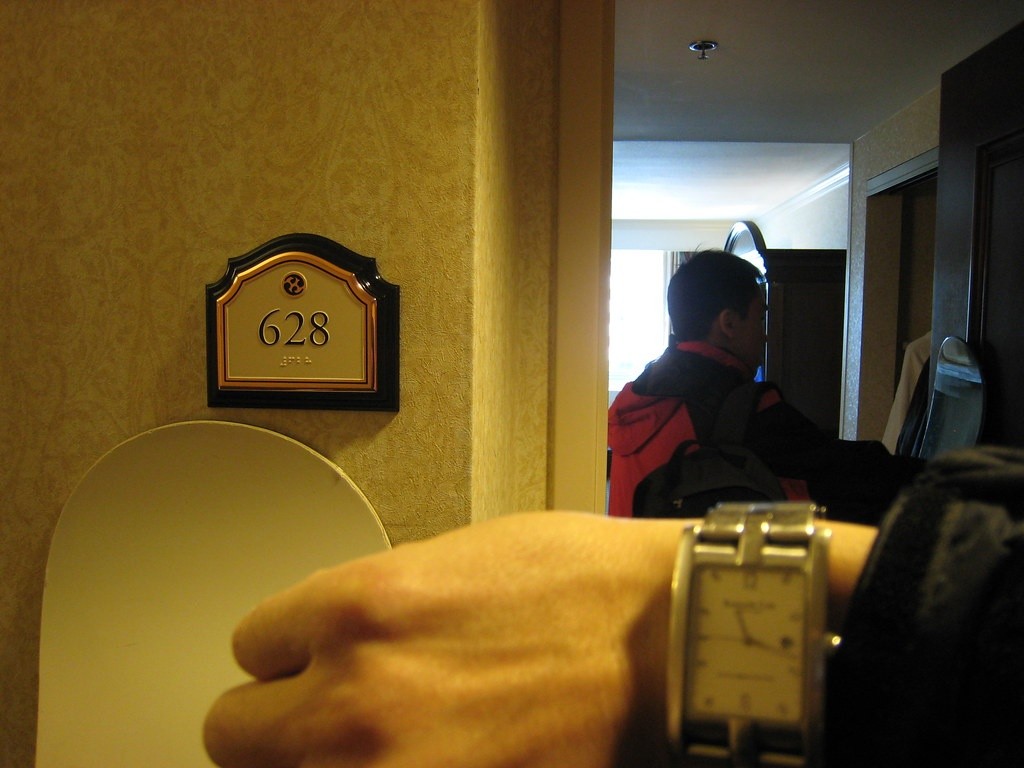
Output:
[663,500,843,768]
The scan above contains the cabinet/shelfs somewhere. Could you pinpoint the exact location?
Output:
[723,223,847,440]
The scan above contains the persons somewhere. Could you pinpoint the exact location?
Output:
[202,249,1024,768]
